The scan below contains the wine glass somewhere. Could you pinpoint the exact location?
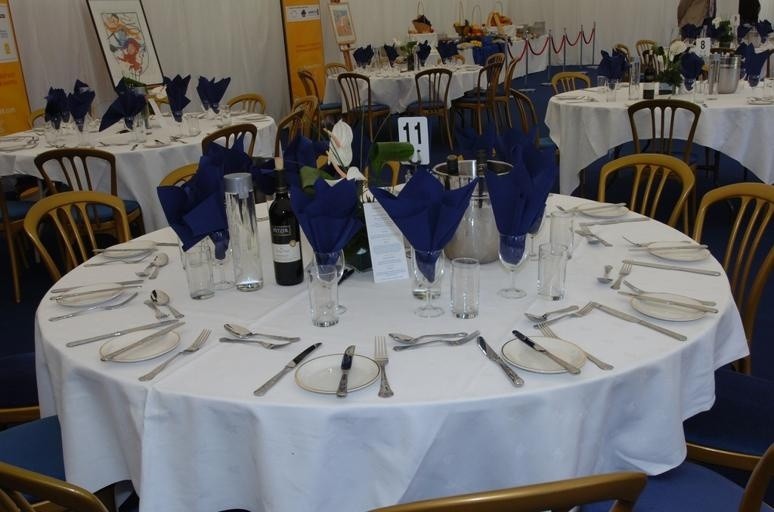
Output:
[406,245,445,320]
[312,250,347,315]
[207,235,234,291]
[495,231,527,300]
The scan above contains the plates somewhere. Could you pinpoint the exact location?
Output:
[646,241,710,262]
[584,89,596,92]
[231,110,246,116]
[579,203,627,218]
[102,238,155,258]
[623,259,724,277]
[245,115,265,120]
[554,93,583,100]
[98,326,178,363]
[56,282,121,308]
[295,353,381,395]
[0,135,39,152]
[501,335,587,372]
[632,291,708,322]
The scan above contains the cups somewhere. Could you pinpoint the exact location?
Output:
[594,73,640,104]
[306,266,340,328]
[350,60,462,77]
[536,241,566,301]
[550,209,573,260]
[449,258,482,319]
[177,232,215,300]
[690,75,774,105]
[43,103,232,150]
[406,248,442,299]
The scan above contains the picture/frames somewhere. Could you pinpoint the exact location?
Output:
[85,0,167,90]
[327,1,360,45]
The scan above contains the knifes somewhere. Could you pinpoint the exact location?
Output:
[476,335,525,386]
[48,280,143,300]
[333,345,356,398]
[591,299,689,343]
[578,217,649,226]
[66,319,177,348]
[629,244,708,252]
[254,341,322,396]
[90,248,158,252]
[511,328,580,376]
[100,321,183,363]
[581,202,626,211]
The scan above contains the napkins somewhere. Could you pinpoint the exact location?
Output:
[274,103,392,168]
[398,100,562,262]
[289,177,367,264]
[371,165,480,285]
[154,126,314,261]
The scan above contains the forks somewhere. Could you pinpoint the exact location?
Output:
[373,334,395,398]
[623,235,647,247]
[609,255,632,292]
[84,253,151,267]
[47,292,137,322]
[219,335,293,350]
[537,301,594,328]
[138,326,211,382]
[618,280,718,315]
[135,255,157,278]
[539,323,617,370]
[142,298,170,320]
[393,329,482,352]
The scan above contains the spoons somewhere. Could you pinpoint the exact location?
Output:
[149,252,170,280]
[387,329,469,346]
[151,288,185,319]
[521,305,579,322]
[596,263,613,286]
[223,322,301,343]
[573,226,613,247]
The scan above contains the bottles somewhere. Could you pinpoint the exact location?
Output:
[220,171,264,293]
[444,153,460,175]
[475,148,486,175]
[642,54,655,99]
[267,156,304,287]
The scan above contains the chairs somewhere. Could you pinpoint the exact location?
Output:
[157,163,201,192]
[599,152,696,232]
[494,58,528,129]
[591,438,774,510]
[33,146,143,242]
[22,190,130,280]
[296,68,341,119]
[335,73,392,144]
[291,95,319,140]
[324,62,348,76]
[692,182,774,376]
[551,72,591,94]
[271,109,305,169]
[0,461,112,512]
[201,123,258,166]
[29,108,47,128]
[372,468,650,511]
[486,52,505,86]
[363,37,549,81]
[406,68,455,152]
[0,186,46,304]
[627,98,702,158]
[226,93,266,117]
[613,37,774,81]
[454,62,502,136]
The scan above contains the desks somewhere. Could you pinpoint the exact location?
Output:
[329,61,483,115]
[546,80,774,186]
[33,192,739,512]
[1,111,276,237]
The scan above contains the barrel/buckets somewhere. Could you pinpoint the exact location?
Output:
[379,43,408,73]
[126,85,148,132]
[708,54,742,95]
[432,158,514,265]
